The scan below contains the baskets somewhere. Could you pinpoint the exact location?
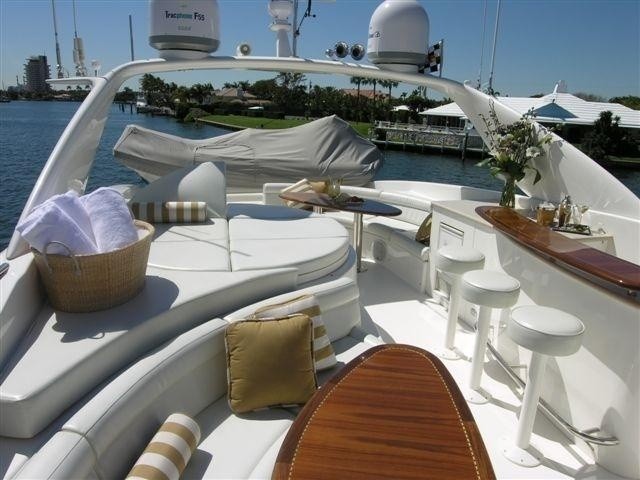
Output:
[29,207,155,314]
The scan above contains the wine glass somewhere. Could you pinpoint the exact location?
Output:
[560,199,593,231]
[324,177,341,207]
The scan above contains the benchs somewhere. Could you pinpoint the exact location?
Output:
[13,278,384,480]
[262,183,432,292]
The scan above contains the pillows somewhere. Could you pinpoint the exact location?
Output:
[279,177,313,210]
[224,293,339,415]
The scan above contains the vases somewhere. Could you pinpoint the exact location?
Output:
[499,176,516,208]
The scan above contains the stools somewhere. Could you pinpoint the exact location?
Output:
[436,243,585,467]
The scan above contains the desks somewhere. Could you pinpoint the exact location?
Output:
[278,191,402,273]
[270,344,496,480]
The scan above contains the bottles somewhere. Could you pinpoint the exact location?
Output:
[559,195,572,227]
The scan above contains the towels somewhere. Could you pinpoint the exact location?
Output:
[16,187,138,254]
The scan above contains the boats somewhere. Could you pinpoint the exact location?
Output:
[0,0,640,480]
[134,94,181,118]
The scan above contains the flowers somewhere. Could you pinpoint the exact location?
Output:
[478,100,555,206]
